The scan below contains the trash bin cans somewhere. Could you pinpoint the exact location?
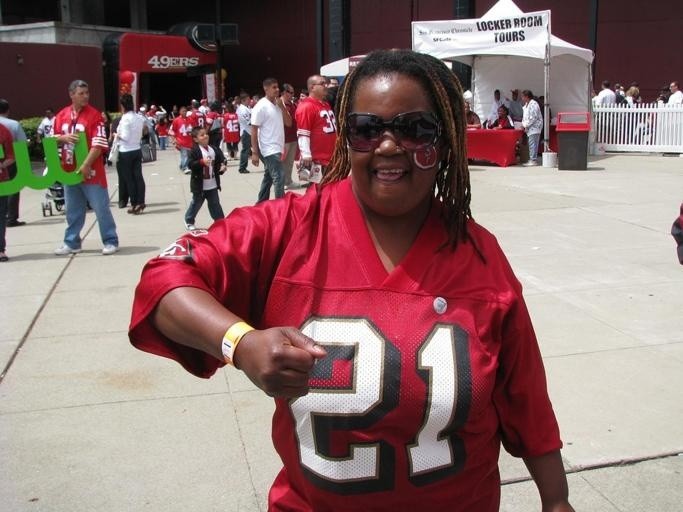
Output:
[555,112,592,170]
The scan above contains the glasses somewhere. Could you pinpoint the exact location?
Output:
[313,82,327,86]
[345,112,445,152]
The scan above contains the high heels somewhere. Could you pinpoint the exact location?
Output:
[127,204,146,214]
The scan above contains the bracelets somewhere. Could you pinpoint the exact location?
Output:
[220,321,254,370]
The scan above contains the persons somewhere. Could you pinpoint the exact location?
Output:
[126,47,574,512]
[2,73,348,258]
[456,76,683,170]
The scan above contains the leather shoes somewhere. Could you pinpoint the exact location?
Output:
[0,257,8,261]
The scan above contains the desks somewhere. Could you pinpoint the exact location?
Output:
[538,125,558,156]
[464,127,524,167]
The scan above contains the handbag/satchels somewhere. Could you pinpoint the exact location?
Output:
[141,143,156,162]
[209,119,221,132]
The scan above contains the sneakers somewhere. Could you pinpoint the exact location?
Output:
[184,169,192,174]
[184,219,196,230]
[119,200,126,208]
[523,160,538,166]
[55,243,81,255]
[102,244,119,254]
[6,219,26,226]
[239,169,250,173]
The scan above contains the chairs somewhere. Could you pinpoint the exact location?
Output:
[510,115,522,156]
